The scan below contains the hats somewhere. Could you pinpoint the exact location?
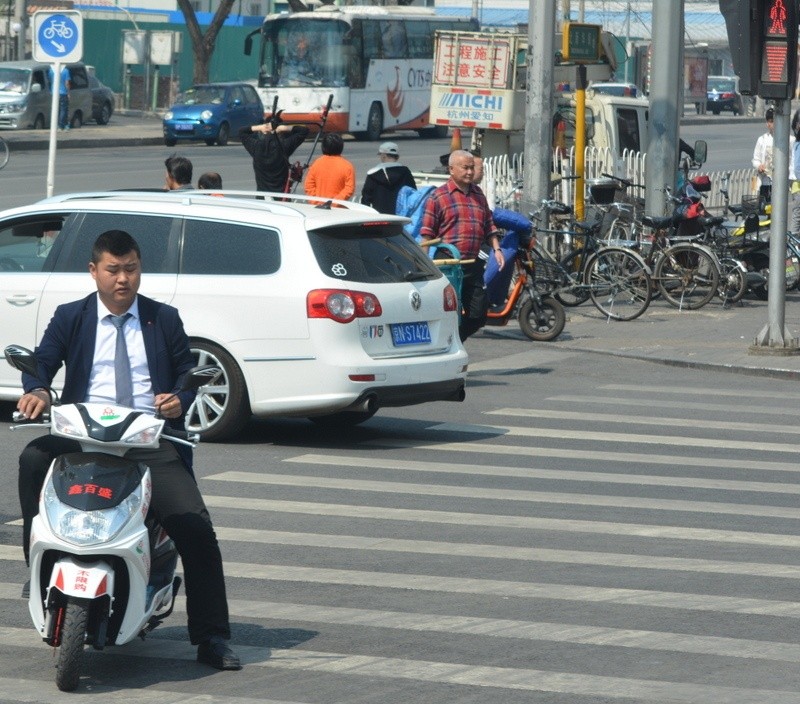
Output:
[376,143,398,155]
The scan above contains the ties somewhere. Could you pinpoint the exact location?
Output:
[107,313,133,407]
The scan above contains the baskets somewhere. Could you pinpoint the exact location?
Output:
[741,196,766,220]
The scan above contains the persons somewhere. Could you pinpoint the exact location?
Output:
[303,133,356,207]
[469,150,508,308]
[752,108,795,228]
[197,171,226,197]
[420,150,505,345]
[163,152,194,190]
[16,230,241,671]
[361,142,417,215]
[789,109,800,234]
[49,64,71,133]
[238,116,309,203]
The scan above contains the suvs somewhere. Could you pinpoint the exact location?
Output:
[0,188,472,443]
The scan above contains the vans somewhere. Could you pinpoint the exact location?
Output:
[0,59,93,130]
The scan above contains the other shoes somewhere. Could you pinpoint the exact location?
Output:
[22,580,29,599]
[197,642,240,671]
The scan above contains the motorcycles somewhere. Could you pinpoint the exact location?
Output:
[653,140,800,300]
[395,186,567,342]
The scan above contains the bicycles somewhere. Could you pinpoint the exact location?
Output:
[497,172,749,323]
[716,172,800,263]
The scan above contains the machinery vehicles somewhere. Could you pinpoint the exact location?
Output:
[428,29,651,182]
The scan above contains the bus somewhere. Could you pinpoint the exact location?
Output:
[242,5,480,138]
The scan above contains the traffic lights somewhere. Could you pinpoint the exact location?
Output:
[560,22,603,65]
[754,0,799,98]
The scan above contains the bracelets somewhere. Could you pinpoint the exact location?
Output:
[493,248,501,253]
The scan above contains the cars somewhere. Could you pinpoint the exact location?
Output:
[91,76,116,126]
[163,82,266,147]
[695,75,742,117]
[585,82,648,100]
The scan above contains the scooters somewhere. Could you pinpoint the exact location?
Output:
[4,344,224,693]
